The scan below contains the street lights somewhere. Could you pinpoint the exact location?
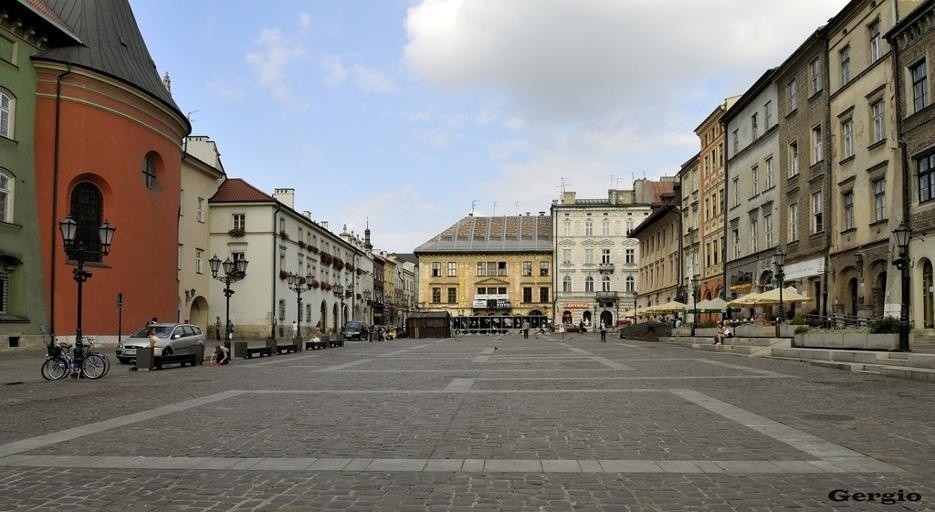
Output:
[615,297,620,327]
[332,281,355,335]
[362,287,381,342]
[633,291,638,325]
[892,221,913,352]
[286,269,315,336]
[773,247,788,325]
[208,252,250,360]
[58,213,116,378]
[690,276,700,337]
[594,305,598,328]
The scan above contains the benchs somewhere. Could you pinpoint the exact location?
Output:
[243,347,272,358]
[276,344,298,354]
[306,342,326,350]
[329,341,342,347]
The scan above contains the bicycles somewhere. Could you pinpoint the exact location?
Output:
[46,342,110,379]
[41,338,105,381]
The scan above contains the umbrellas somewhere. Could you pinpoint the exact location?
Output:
[625,287,816,320]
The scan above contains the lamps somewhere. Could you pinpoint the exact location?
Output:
[855,254,863,263]
[185,288,195,298]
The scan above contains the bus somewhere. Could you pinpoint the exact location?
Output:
[451,315,550,335]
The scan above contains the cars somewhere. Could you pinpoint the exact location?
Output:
[339,320,369,341]
[370,324,406,338]
[552,323,588,334]
[116,322,207,367]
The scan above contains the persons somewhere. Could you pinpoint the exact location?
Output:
[145,317,160,335]
[290,320,298,338]
[213,316,221,340]
[210,344,230,366]
[228,319,234,341]
[377,314,733,346]
[128,330,161,371]
